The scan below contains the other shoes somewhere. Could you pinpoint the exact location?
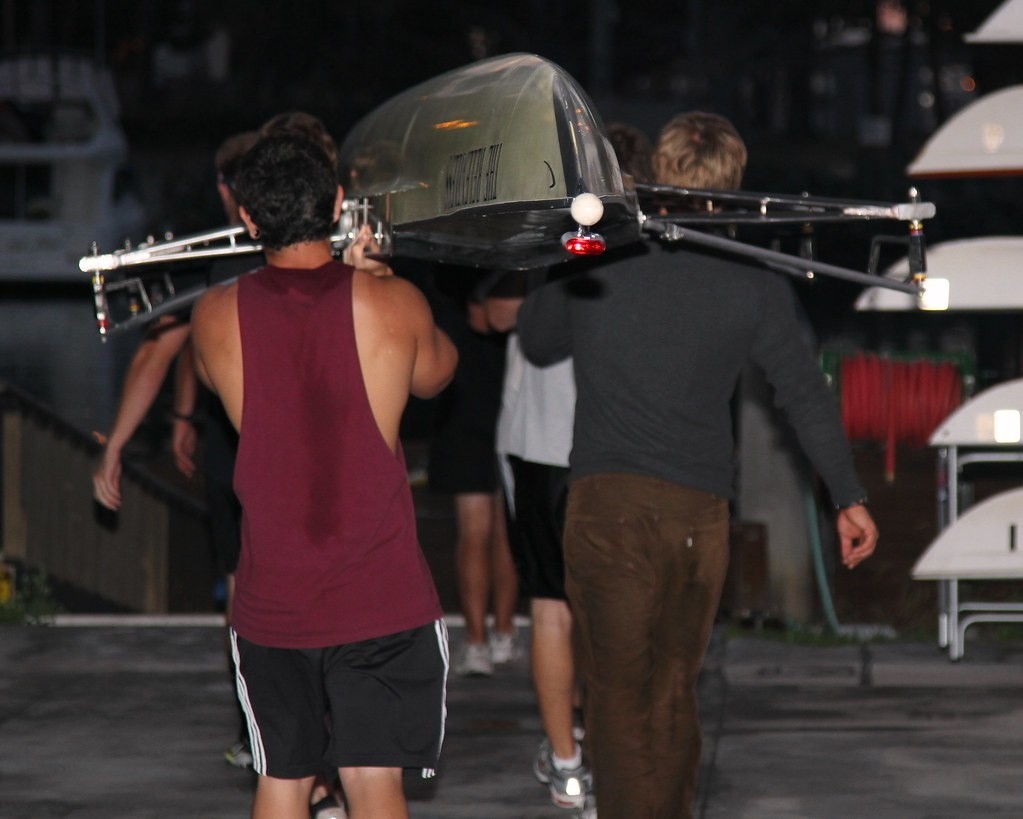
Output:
[457,649,493,674]
[489,633,525,662]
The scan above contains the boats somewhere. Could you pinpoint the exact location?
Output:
[75,49,940,348]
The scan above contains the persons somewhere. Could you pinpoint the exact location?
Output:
[83,115,459,819]
[457,104,880,819]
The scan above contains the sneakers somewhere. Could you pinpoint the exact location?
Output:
[533,738,585,808]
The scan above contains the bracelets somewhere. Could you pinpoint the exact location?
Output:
[174,409,194,423]
